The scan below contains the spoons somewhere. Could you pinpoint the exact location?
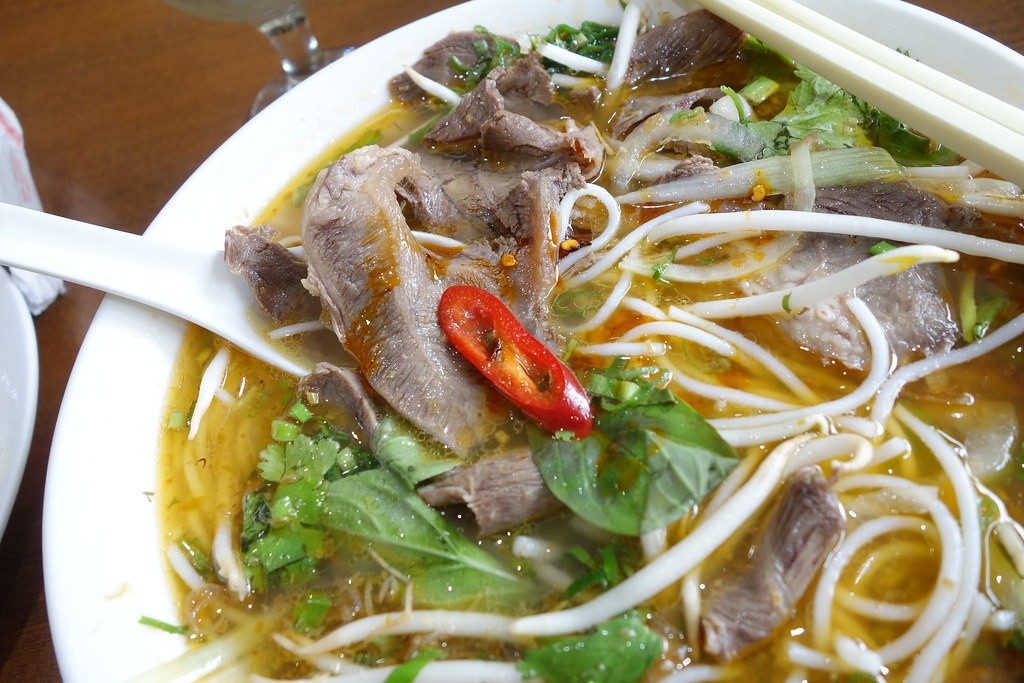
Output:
[0,203,466,378]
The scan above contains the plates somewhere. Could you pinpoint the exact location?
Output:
[41,0,1024,683]
[0,267,40,540]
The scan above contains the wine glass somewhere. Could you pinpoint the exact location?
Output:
[169,0,358,122]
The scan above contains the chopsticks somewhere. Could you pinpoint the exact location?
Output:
[697,0,1024,190]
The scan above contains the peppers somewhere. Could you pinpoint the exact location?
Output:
[436,285,593,442]
